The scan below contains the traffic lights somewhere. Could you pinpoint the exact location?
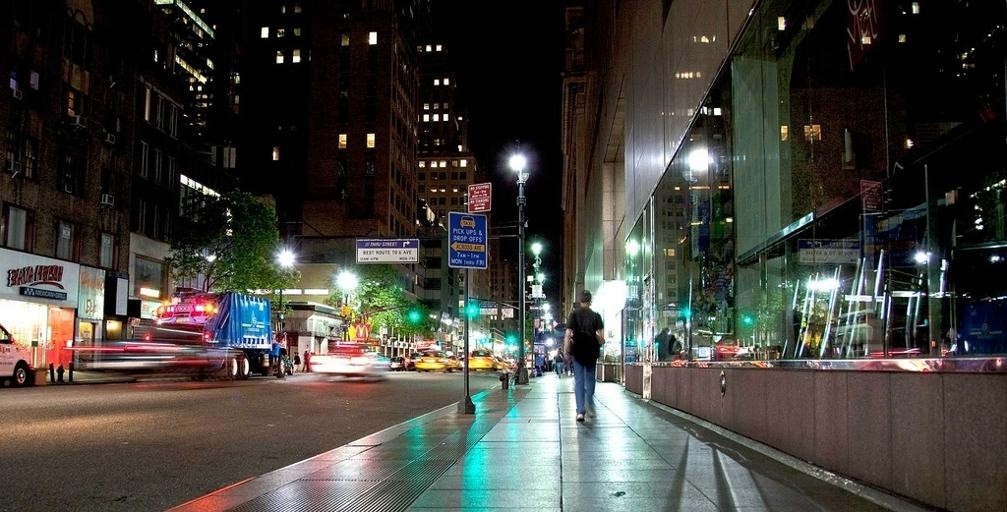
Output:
[468,301,477,321]
[410,309,417,323]
[930,339,936,349]
[507,335,515,344]
[479,335,486,344]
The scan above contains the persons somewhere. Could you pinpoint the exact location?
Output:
[654,327,678,362]
[543,348,576,378]
[564,290,604,422]
[294,350,312,374]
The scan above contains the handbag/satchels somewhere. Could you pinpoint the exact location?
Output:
[565,335,573,355]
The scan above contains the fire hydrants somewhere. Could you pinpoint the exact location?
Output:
[499,368,509,389]
[56,364,65,382]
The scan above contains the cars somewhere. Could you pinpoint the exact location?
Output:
[945,297,1005,355]
[684,333,738,360]
[390,347,516,373]
[945,343,957,357]
[309,343,390,379]
[0,323,31,386]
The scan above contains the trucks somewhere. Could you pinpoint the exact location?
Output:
[143,291,293,379]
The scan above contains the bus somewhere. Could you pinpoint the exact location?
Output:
[827,310,883,358]
[938,239,1007,355]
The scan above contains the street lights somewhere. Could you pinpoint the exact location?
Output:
[531,242,543,378]
[508,137,529,386]
[537,272,557,376]
[274,247,294,332]
[444,317,452,351]
[685,145,711,360]
[336,269,356,342]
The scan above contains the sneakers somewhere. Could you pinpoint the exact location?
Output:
[576,414,584,420]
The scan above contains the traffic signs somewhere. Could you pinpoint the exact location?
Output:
[861,180,884,211]
[355,238,419,264]
[478,303,497,315]
[798,236,859,265]
[858,207,929,272]
[447,211,487,270]
[467,183,491,212]
